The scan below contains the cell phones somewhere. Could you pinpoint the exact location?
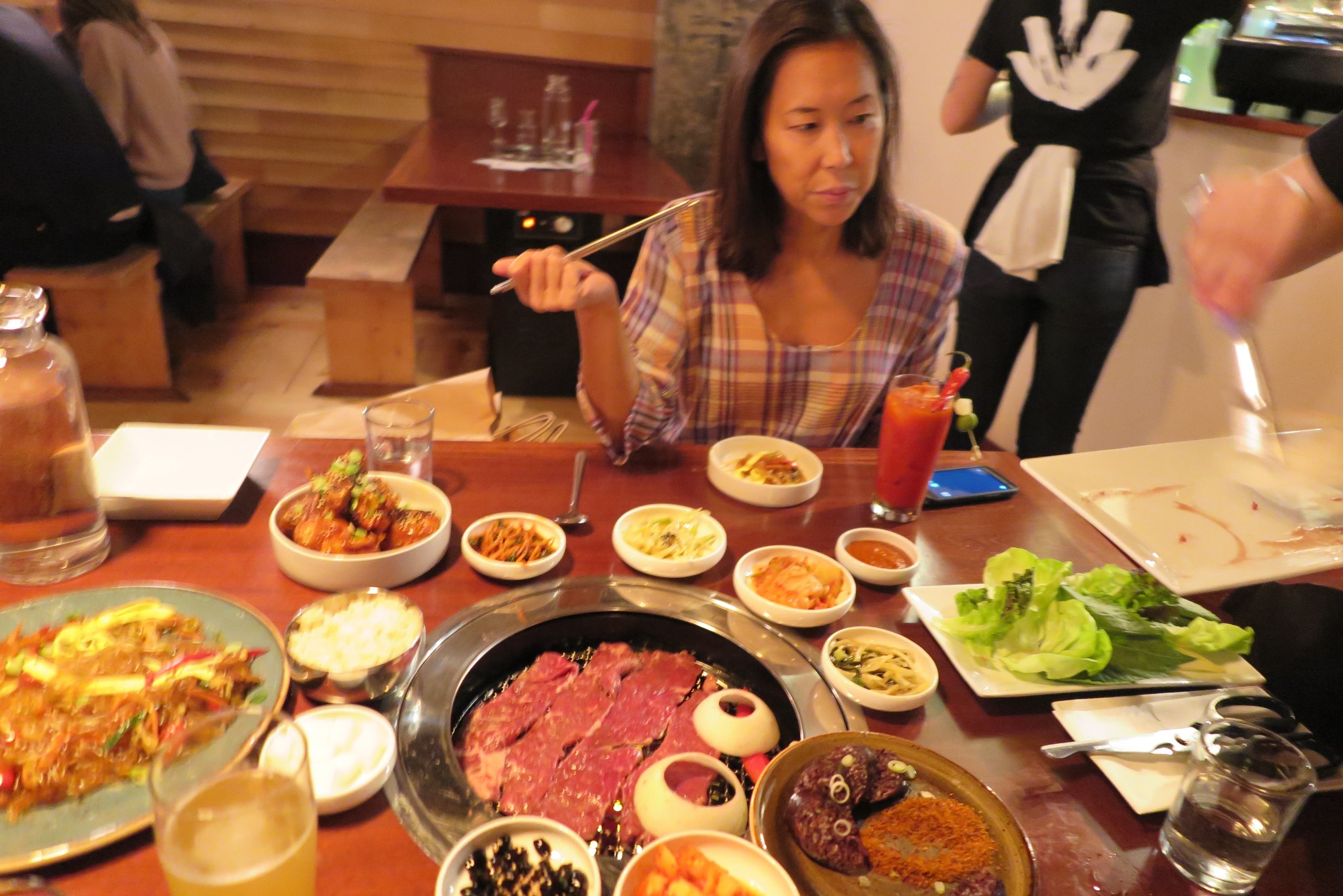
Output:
[918,465,1019,508]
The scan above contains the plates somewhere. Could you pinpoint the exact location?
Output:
[90,417,272,520]
[1040,697,1343,815]
[1013,424,1343,592]
[751,727,1037,896]
[901,583,1267,697]
[3,582,289,875]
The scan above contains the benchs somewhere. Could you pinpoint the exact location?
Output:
[4,175,253,389]
[305,189,443,385]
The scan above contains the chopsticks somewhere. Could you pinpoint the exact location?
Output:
[487,203,703,297]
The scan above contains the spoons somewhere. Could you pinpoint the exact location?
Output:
[553,449,589,526]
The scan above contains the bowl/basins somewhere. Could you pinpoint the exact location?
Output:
[709,435,824,504]
[462,513,565,580]
[253,706,399,812]
[733,542,857,627]
[432,816,603,896]
[838,526,921,585]
[267,474,454,592]
[274,587,425,706]
[827,626,937,713]
[608,832,795,896]
[612,503,724,578]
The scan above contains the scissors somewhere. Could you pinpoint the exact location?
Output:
[1040,694,1340,780]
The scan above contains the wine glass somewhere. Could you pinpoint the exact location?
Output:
[483,97,509,161]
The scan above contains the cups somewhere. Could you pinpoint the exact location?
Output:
[574,120,598,174]
[144,708,316,896]
[364,398,436,486]
[517,109,537,157]
[870,373,953,522]
[1160,721,1316,896]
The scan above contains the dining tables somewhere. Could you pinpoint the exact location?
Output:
[384,111,696,398]
[1,421,1341,896]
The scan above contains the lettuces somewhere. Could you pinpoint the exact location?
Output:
[954,546,1254,684]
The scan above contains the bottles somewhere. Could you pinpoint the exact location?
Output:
[2,280,112,587]
[541,72,570,166]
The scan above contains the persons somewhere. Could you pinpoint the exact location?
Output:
[1178,110,1343,327]
[53,0,194,211]
[1,3,151,267]
[937,0,1248,457]
[490,0,970,471]
